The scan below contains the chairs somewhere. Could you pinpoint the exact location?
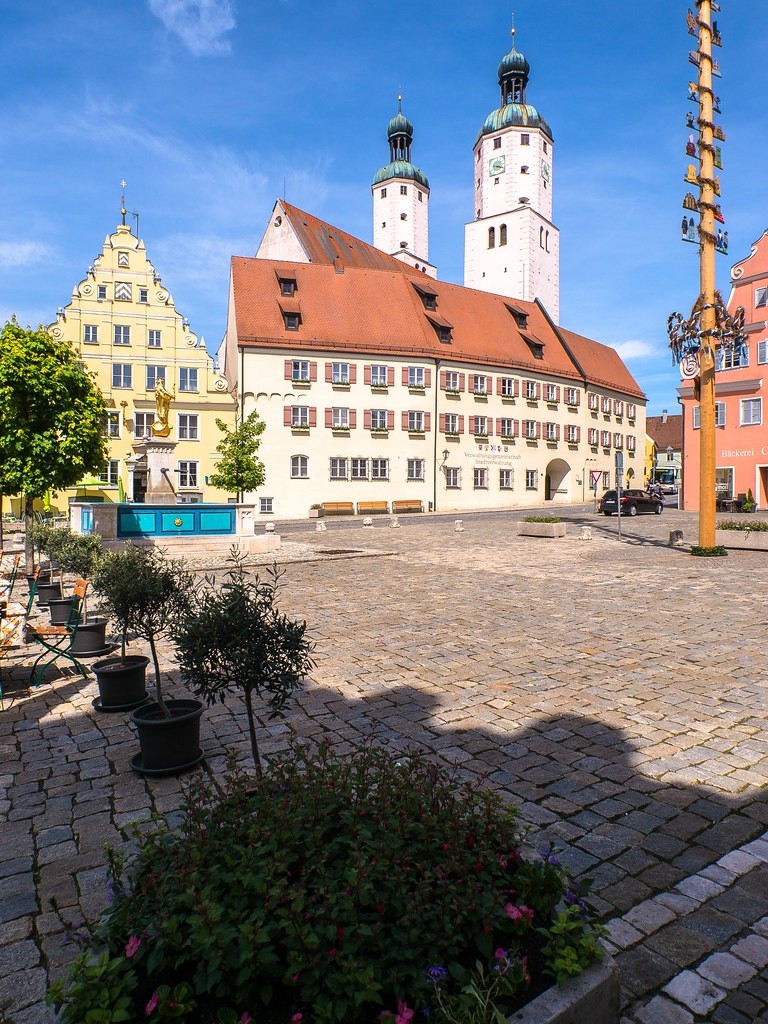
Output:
[0,554,20,603]
[22,576,90,688]
[0,564,41,654]
[733,493,747,512]
[716,493,726,512]
[2,510,66,527]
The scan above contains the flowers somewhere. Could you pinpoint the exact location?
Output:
[71,743,596,1024]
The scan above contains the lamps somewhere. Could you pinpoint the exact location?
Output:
[439,448,449,469]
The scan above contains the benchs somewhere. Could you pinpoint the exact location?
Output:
[391,500,423,513]
[356,501,390,514]
[321,502,353,515]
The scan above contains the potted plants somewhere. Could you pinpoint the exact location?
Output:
[517,515,566,536]
[25,518,113,657]
[308,504,323,517]
[714,519,767,549]
[742,487,756,513]
[90,540,151,712]
[113,545,206,777]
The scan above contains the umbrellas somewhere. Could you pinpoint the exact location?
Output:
[44,475,125,526]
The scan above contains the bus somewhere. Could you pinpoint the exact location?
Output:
[652,466,679,495]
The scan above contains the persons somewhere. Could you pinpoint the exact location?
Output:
[155,384,174,424]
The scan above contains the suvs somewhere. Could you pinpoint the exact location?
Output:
[600,489,664,516]
[716,491,732,511]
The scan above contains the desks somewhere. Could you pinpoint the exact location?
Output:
[722,499,738,512]
[3,516,16,523]
[53,515,68,525]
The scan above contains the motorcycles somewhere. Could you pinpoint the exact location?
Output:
[652,491,666,501]
